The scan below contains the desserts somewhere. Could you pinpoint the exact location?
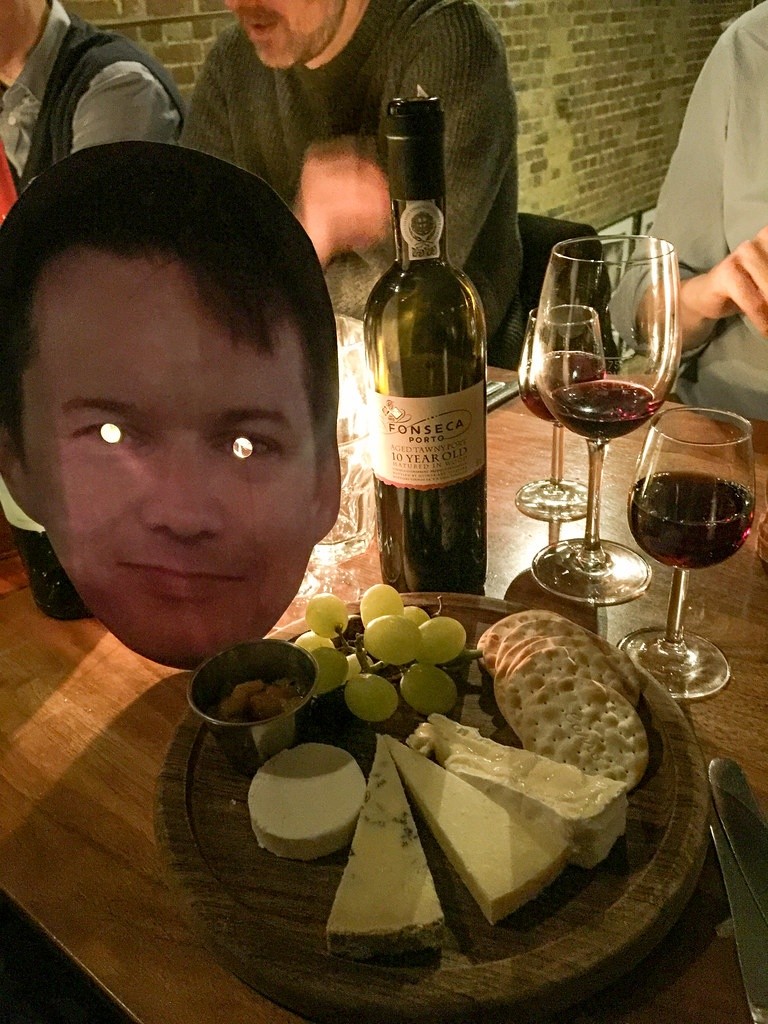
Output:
[247,713,628,955]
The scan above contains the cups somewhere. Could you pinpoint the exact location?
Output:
[186,640,321,771]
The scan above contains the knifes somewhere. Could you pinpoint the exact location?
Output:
[706,755,767,1023]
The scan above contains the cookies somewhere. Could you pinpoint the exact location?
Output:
[476,609,649,794]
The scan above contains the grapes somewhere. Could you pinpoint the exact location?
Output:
[291,582,467,722]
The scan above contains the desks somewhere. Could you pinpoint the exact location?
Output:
[0,363,768,1024]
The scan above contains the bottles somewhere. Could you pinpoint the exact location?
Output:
[362,93,489,597]
[1,463,94,622]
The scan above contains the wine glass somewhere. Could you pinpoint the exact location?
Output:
[267,312,378,638]
[518,236,756,702]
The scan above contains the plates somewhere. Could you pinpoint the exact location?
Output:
[149,593,708,1024]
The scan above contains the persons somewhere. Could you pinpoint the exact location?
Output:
[0,0,186,193]
[0,138,343,670]
[179,0,526,372]
[608,0,768,424]
